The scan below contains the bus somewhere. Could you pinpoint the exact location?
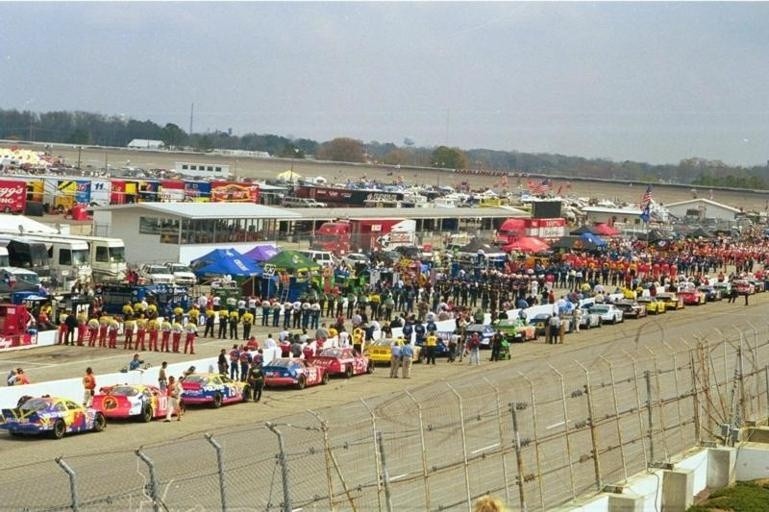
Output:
[0,230,126,291]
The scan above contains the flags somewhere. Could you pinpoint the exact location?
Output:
[640,187,653,214]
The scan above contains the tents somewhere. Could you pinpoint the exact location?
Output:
[190,224,614,283]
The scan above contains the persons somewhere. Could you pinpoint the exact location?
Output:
[7,273,267,423]
[443,247,602,365]
[591,237,769,317]
[245,263,448,379]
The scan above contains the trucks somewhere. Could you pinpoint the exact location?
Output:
[495,219,566,245]
[308,219,416,255]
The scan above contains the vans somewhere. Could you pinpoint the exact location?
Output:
[99,284,156,313]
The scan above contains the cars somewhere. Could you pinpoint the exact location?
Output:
[292,246,503,283]
[261,357,329,389]
[363,338,425,366]
[0,397,106,438]
[181,373,252,408]
[164,262,197,285]
[92,383,186,422]
[150,286,191,303]
[139,263,175,285]
[312,347,374,378]
